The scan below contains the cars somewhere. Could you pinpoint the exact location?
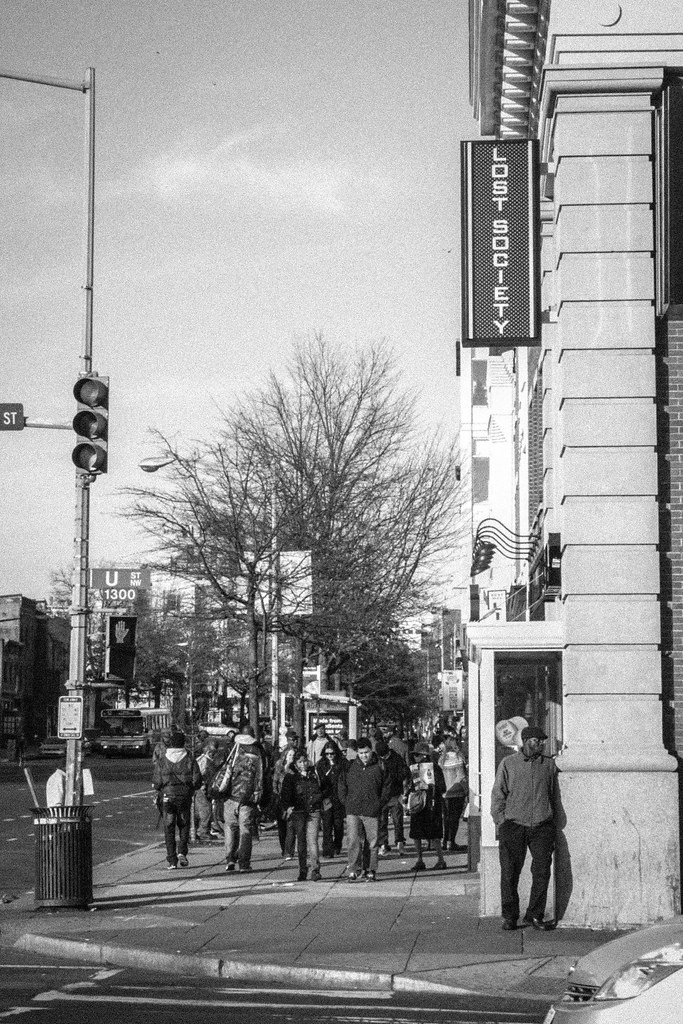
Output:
[198,721,241,738]
[38,735,93,758]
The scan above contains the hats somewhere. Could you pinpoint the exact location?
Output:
[410,743,431,756]
[313,722,325,729]
[285,731,299,738]
[521,727,548,740]
[375,743,389,756]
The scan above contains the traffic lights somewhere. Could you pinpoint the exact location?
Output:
[102,618,137,683]
[68,376,109,478]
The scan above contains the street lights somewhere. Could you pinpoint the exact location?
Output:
[137,456,282,753]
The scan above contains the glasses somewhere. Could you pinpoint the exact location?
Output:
[325,753,334,755]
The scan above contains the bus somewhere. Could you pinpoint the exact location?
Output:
[99,708,172,758]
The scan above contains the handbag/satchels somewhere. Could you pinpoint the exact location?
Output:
[210,744,239,797]
[407,791,427,815]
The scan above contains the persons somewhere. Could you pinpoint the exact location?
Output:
[282,750,327,882]
[491,726,556,930]
[151,727,467,871]
[337,738,390,882]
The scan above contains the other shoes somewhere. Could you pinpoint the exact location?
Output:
[397,841,404,856]
[177,851,189,866]
[502,918,516,930]
[524,914,548,932]
[447,841,467,851]
[311,870,321,881]
[348,871,356,882]
[199,832,216,841]
[412,862,425,873]
[240,866,253,873]
[378,846,392,856]
[225,861,235,871]
[298,872,307,882]
[167,864,177,870]
[365,873,375,883]
[285,854,292,861]
[431,861,447,870]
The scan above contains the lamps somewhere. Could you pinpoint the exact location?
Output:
[471,518,541,578]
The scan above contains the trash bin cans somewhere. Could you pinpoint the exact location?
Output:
[31,804,94,912]
